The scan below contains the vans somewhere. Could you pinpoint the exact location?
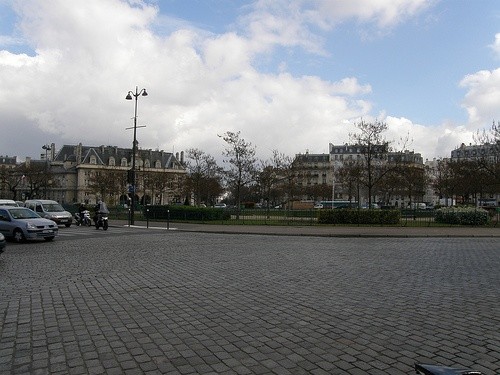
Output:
[406,202,426,211]
[23,199,75,226]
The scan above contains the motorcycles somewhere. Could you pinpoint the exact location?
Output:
[93,201,110,230]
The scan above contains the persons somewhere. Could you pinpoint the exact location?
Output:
[79,204,86,223]
[94,200,103,228]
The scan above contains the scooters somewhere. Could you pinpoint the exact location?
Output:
[73,207,92,227]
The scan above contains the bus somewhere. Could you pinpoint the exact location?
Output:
[320,200,358,210]
[475,198,499,207]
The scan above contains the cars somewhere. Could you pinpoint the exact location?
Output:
[0,199,59,249]
[211,203,236,209]
[199,203,208,207]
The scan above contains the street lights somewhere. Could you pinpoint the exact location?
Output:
[42,144,52,199]
[125,85,148,226]
